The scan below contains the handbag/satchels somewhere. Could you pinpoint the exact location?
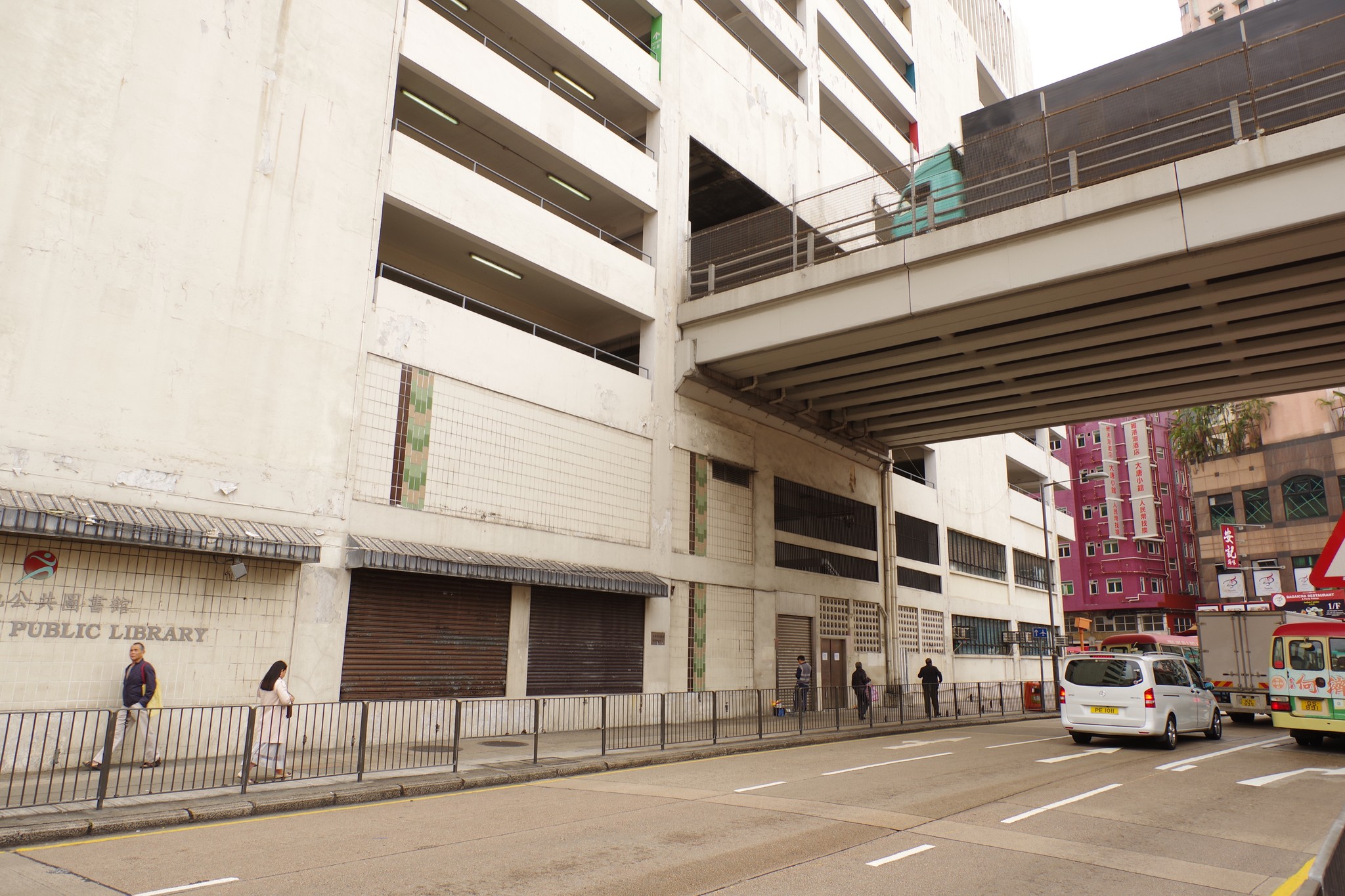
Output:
[865,680,879,701]
[141,662,163,716]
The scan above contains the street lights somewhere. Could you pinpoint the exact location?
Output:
[1039,471,1110,710]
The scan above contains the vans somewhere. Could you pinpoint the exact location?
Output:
[1268,622,1345,747]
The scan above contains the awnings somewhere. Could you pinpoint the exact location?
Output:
[0,488,321,563]
[345,534,668,598]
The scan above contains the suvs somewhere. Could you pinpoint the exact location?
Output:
[1059,650,1222,750]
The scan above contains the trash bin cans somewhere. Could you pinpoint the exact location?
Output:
[1025,681,1042,711]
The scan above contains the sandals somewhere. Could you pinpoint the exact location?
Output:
[83,760,101,770]
[140,758,162,769]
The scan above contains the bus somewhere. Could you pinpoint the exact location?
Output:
[1100,633,1201,678]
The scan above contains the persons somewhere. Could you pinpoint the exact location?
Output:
[791,655,812,717]
[918,658,942,717]
[82,643,161,768]
[237,661,294,783]
[852,662,871,721]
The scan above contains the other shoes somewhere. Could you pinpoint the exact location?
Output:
[926,713,929,717]
[238,771,254,783]
[935,713,941,717]
[859,712,865,719]
[275,772,292,778]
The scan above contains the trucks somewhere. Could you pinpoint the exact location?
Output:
[1194,610,1343,726]
